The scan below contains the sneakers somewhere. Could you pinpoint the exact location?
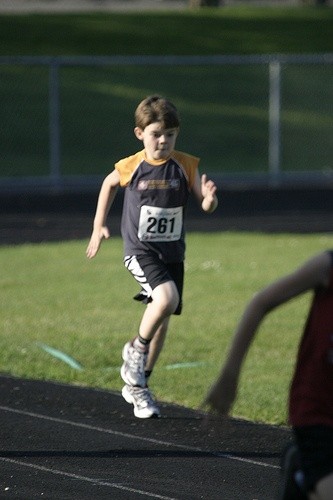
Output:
[121,384,160,418]
[120,335,145,388]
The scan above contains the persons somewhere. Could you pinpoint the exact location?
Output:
[86,94,218,418]
[207,252,333,500]
